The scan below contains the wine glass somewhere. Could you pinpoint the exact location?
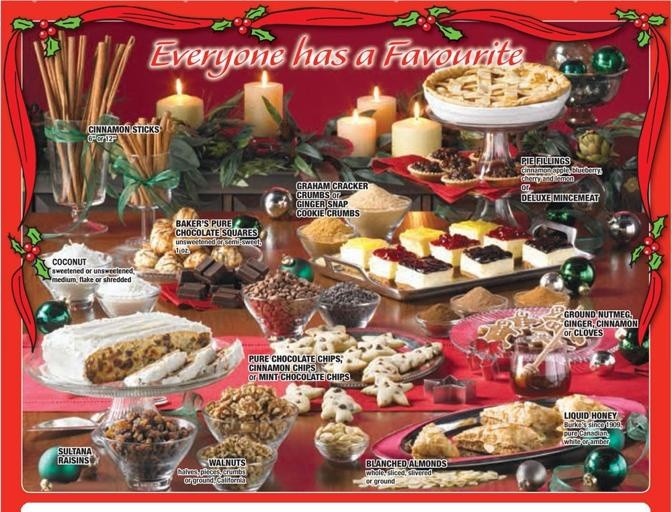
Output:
[44,111,119,236]
[120,150,173,251]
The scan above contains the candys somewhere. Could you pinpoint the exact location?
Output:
[132,208,269,307]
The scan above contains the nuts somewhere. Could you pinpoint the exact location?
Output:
[315,422,366,451]
[104,412,189,481]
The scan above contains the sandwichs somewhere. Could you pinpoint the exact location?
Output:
[412,423,461,461]
[454,424,541,458]
[480,401,563,434]
[556,393,613,432]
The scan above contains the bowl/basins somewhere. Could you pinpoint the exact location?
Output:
[33,254,113,310]
[320,294,381,328]
[243,297,320,338]
[513,294,570,310]
[93,417,198,491]
[417,315,461,337]
[197,445,277,493]
[314,438,369,463]
[451,296,509,317]
[298,226,358,265]
[337,196,413,239]
[201,399,299,452]
[95,285,160,318]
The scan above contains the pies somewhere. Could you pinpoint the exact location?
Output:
[172,332,210,350]
[85,334,171,384]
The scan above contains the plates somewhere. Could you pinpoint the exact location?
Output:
[371,395,646,468]
[310,221,596,300]
[110,242,264,284]
[421,91,570,124]
[300,327,444,386]
[450,306,625,362]
[24,338,238,445]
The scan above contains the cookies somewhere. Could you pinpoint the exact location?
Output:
[270,323,446,420]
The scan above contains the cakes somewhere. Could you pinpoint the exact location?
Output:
[484,226,529,259]
[431,234,481,268]
[461,244,515,279]
[449,219,502,240]
[341,237,387,270]
[369,247,417,278]
[396,255,454,288]
[520,237,576,269]
[399,227,443,259]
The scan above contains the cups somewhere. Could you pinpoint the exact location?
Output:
[509,346,571,401]
